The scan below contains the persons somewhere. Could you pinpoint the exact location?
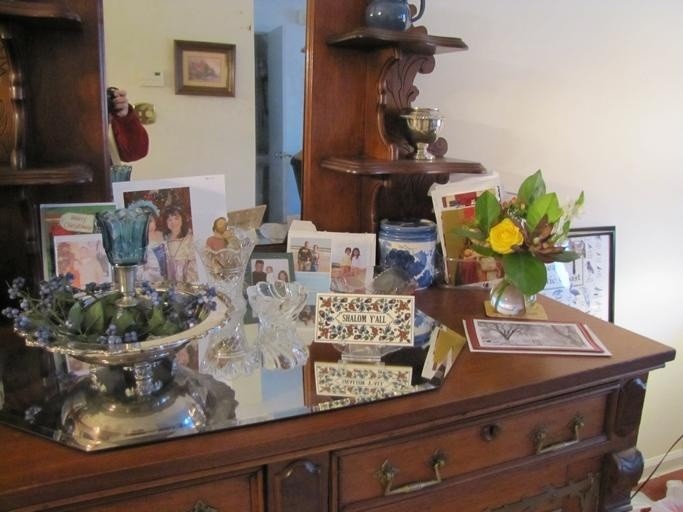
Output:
[310,243,321,272]
[252,257,268,284]
[340,246,351,275]
[278,270,290,284]
[297,240,312,271]
[128,199,167,283]
[264,264,276,284]
[206,215,228,250]
[162,203,199,284]
[351,246,368,270]
[104,86,150,177]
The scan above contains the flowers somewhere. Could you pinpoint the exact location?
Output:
[447,170,586,309]
[1,273,218,348]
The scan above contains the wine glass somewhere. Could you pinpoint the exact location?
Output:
[13,282,234,408]
[396,106,443,162]
[23,373,236,448]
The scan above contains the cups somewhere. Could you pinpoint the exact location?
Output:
[366,1,425,32]
[195,234,312,380]
[95,209,151,266]
[379,218,437,290]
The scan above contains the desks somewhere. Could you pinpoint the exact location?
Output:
[0,282,676,512]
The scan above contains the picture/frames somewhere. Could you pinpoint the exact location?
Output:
[540,225,616,323]
[173,39,236,98]
[242,252,295,324]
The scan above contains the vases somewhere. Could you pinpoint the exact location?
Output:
[14,280,235,453]
[489,272,538,317]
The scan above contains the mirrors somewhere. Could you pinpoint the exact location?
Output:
[102,0,306,260]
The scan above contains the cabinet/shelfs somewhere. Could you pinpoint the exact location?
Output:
[0,0,113,268]
[301,0,488,267]
